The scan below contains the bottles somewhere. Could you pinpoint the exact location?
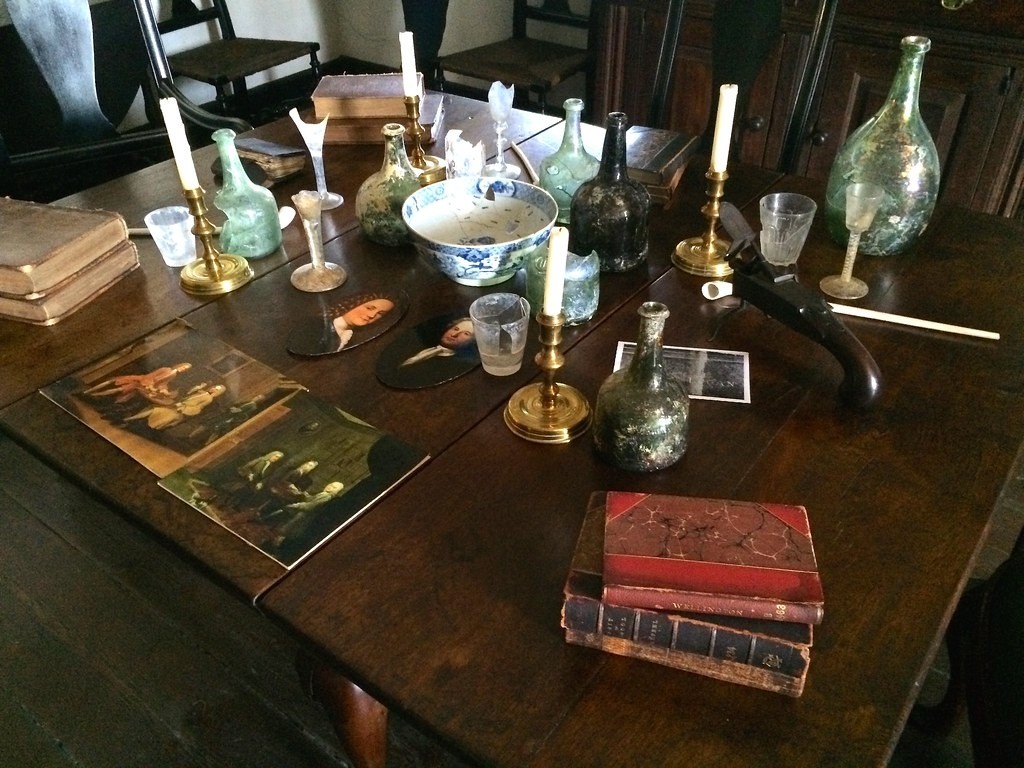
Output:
[353,122,424,247]
[820,35,942,259]
[211,128,284,260]
[567,112,652,274]
[537,98,602,226]
[592,300,691,474]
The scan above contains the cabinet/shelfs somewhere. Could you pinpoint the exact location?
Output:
[598,0,1024,222]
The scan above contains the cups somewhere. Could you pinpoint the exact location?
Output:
[467,293,531,377]
[143,206,198,268]
[758,193,818,266]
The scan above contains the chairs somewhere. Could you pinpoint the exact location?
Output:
[153,0,321,127]
[434,2,593,116]
[1,0,253,200]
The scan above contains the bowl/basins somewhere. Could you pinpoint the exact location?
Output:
[400,175,559,287]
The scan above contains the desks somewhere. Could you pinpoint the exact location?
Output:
[0,89,1024,768]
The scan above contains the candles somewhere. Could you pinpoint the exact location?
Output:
[544,223,568,318]
[159,93,199,193]
[708,84,740,175]
[398,29,419,102]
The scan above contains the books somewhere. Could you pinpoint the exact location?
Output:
[0,197,140,326]
[303,72,446,145]
[561,490,825,697]
[626,125,701,200]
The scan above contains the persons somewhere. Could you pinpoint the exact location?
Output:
[79,361,266,451]
[285,292,401,355]
[375,313,476,390]
[211,451,345,553]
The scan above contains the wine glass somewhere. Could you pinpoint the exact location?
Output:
[821,182,885,300]
[480,80,523,180]
